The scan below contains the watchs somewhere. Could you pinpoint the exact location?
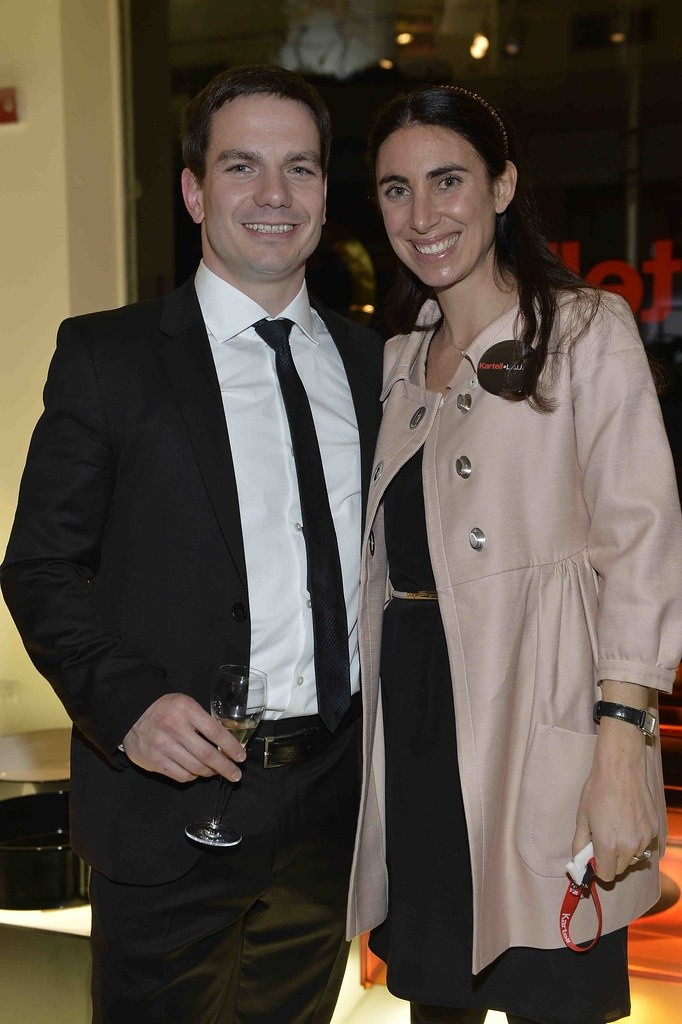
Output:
[593,701,657,746]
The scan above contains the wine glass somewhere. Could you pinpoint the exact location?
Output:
[186,665,267,846]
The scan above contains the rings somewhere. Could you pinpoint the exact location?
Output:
[633,850,652,861]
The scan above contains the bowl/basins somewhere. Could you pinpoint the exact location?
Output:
[0,789,92,910]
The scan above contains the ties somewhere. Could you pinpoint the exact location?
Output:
[253,317,352,732]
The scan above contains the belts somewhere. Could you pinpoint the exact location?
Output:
[244,691,362,768]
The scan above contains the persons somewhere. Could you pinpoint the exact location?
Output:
[1,64,386,1024]
[346,82,682,1024]
